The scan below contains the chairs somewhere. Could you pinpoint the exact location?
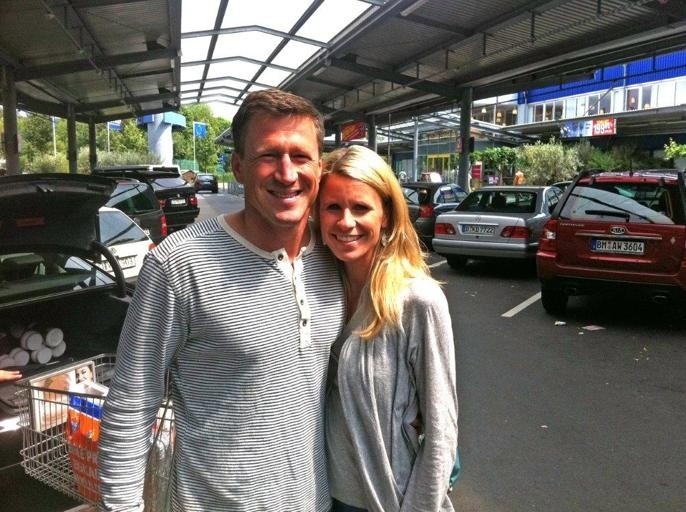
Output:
[489,196,506,210]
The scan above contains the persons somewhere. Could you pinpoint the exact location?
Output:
[76,364,94,384]
[513,168,524,185]
[98,89,425,511]
[0,370,23,384]
[310,144,460,511]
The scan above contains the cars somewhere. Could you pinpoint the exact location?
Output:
[399,181,470,249]
[191,173,218,193]
[60,206,159,292]
[430,186,563,272]
[0,172,140,511]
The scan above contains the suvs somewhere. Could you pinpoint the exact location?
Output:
[535,169,686,315]
[100,170,200,235]
[102,176,168,247]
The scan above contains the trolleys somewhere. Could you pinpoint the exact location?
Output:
[11,352,177,512]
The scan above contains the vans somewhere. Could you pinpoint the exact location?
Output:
[91,164,183,177]
